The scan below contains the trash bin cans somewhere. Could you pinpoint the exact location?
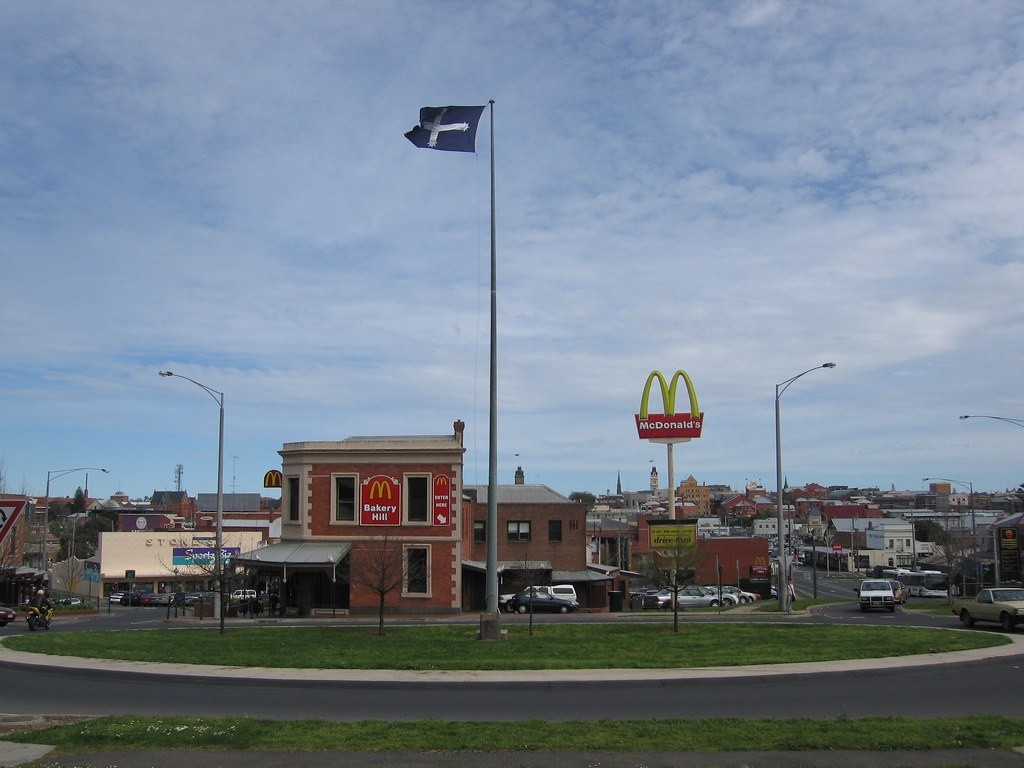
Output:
[607,591,623,611]
[632,596,642,612]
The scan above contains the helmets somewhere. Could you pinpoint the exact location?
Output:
[38,590,43,594]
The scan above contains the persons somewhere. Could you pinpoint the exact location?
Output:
[31,589,52,623]
[787,578,795,612]
[270,593,278,615]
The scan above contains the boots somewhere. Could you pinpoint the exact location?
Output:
[41,612,47,624]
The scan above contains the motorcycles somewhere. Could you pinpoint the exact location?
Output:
[25,598,56,632]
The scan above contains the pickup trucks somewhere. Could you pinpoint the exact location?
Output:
[499,584,580,613]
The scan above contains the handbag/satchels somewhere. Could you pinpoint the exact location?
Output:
[792,594,795,602]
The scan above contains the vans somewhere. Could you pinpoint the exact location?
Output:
[230,589,257,599]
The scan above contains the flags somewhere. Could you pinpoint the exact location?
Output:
[403,106,486,153]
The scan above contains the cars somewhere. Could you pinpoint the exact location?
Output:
[950,587,1024,633]
[510,590,577,615]
[630,584,762,608]
[853,579,896,613]
[0,607,18,627]
[110,591,229,608]
[58,598,82,606]
[883,579,908,604]
[227,597,265,614]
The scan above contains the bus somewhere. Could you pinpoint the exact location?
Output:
[880,569,948,600]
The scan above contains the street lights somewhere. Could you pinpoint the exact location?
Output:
[808,508,830,578]
[160,369,224,619]
[56,514,86,601]
[42,467,109,571]
[881,504,917,572]
[922,476,979,596]
[775,362,839,613]
